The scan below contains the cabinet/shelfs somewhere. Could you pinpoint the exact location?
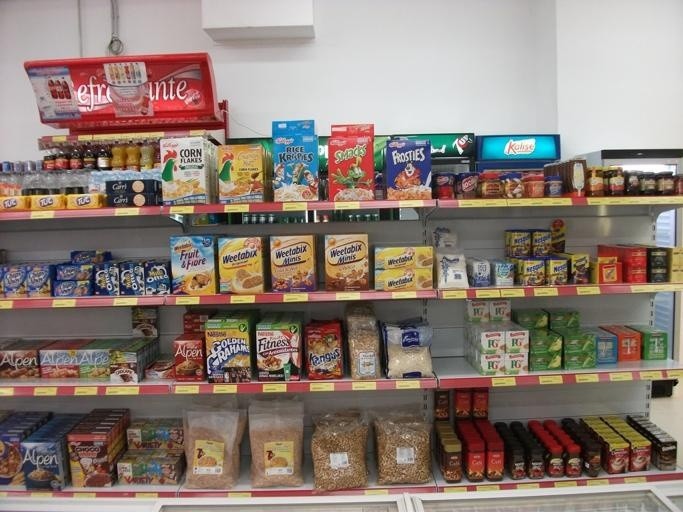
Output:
[2,206,176,499]
[161,199,436,497]
[436,182,683,499]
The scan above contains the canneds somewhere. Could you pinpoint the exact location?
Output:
[434,155,683,198]
[0,160,43,173]
[432,387,677,484]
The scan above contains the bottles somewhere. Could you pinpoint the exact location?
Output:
[44,139,162,194]
